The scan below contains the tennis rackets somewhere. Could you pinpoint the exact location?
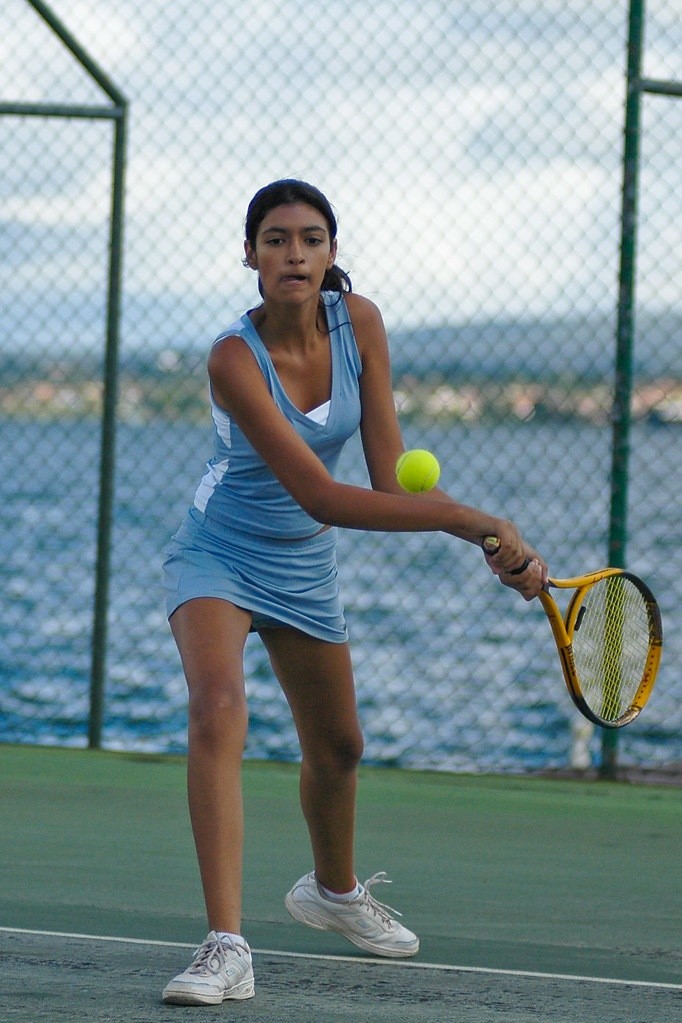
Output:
[482,534,663,729]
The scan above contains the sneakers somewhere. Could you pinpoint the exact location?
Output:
[284,869,421,957]
[160,931,255,1004]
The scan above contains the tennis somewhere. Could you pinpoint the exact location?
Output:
[395,449,441,494]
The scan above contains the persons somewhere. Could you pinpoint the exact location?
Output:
[158,178,550,1004]
